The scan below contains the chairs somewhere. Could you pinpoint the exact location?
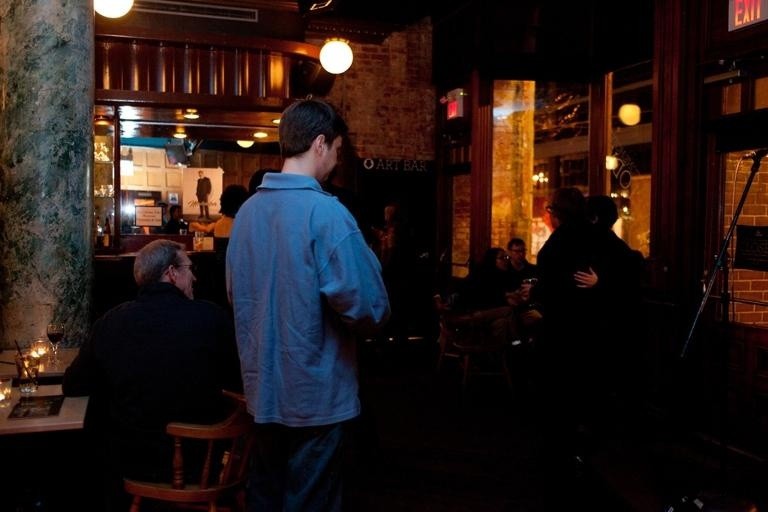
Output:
[125,391,253,510]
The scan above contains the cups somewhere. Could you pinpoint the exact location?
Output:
[194,231,204,251]
[0,377,13,409]
[16,356,40,393]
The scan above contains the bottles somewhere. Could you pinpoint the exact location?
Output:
[94,216,111,249]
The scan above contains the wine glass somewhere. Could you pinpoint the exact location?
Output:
[45,322,65,370]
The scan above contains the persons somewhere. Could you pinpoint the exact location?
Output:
[224,97,397,512]
[154,167,655,375]
[61,238,245,512]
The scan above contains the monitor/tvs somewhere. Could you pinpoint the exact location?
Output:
[135,205,163,234]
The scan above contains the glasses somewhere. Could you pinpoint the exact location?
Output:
[546,204,558,214]
[497,255,511,260]
[164,263,196,274]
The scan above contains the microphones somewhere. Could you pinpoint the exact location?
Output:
[743,149,768,161]
